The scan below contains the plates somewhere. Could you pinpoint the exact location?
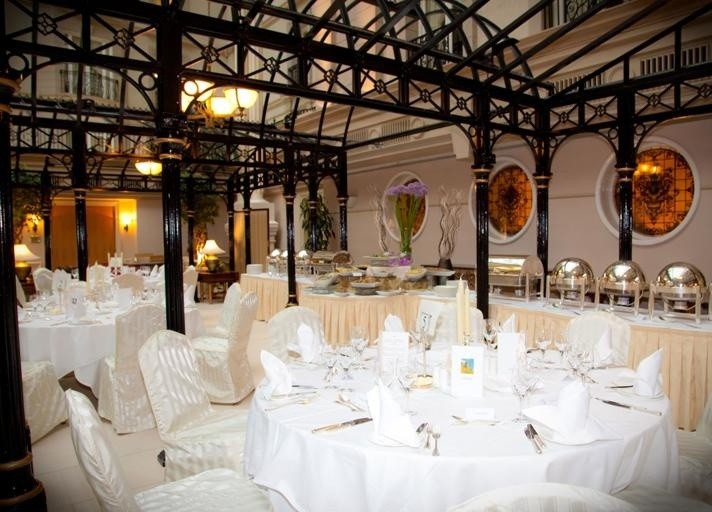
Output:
[375,289,403,296]
[429,269,456,276]
[337,271,356,278]
[312,278,332,285]
[404,287,426,296]
[611,378,665,398]
[246,263,265,274]
[352,282,380,289]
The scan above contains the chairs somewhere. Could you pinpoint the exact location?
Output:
[264,306,323,359]
[674,389,712,494]
[440,304,484,345]
[136,251,153,263]
[32,267,53,296]
[64,389,274,511]
[449,480,637,512]
[211,282,241,339]
[50,269,68,295]
[99,303,165,435]
[183,264,197,308]
[566,313,630,367]
[115,273,145,296]
[20,362,70,444]
[150,255,164,272]
[188,292,260,405]
[138,329,249,483]
[86,264,110,283]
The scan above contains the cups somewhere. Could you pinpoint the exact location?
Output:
[394,354,418,418]
[35,293,45,315]
[378,355,397,418]
[350,326,370,372]
[511,369,535,424]
[535,326,554,373]
[577,350,593,389]
[481,318,500,361]
[322,345,341,392]
[566,343,582,380]
[555,332,569,372]
[29,294,40,318]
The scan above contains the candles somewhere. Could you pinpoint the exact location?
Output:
[456,274,471,344]
[114,252,118,272]
[101,268,106,293]
[94,259,99,294]
[85,264,91,293]
[107,252,112,272]
[120,252,123,272]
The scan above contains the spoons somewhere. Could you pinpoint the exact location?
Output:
[433,429,441,458]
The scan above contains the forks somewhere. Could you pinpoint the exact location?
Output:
[658,315,698,328]
[422,423,432,452]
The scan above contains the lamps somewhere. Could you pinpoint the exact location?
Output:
[199,239,225,271]
[120,213,131,232]
[14,244,41,282]
[27,214,41,244]
[205,95,233,122]
[134,159,163,176]
[223,86,259,113]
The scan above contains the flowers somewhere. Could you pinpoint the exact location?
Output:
[386,182,430,266]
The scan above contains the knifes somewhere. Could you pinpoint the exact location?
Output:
[527,424,548,452]
[312,417,374,436]
[593,395,663,418]
[522,426,541,454]
[413,422,428,435]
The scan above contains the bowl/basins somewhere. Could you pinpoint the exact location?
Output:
[433,285,462,297]
[405,269,426,282]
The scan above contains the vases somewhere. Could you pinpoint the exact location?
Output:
[399,254,411,268]
